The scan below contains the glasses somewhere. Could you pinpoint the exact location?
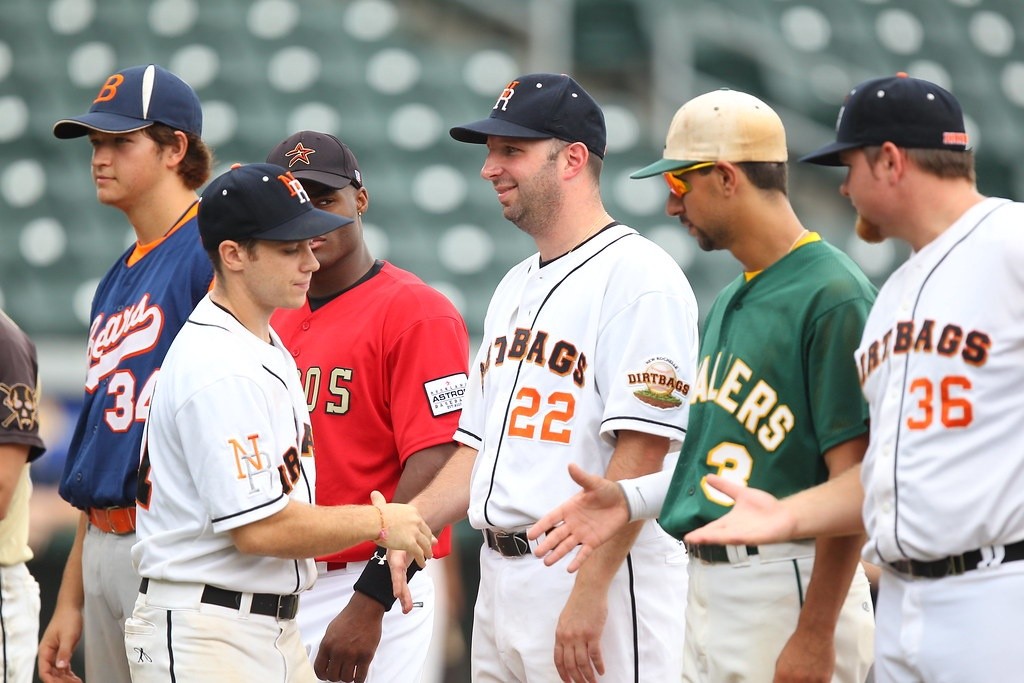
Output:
[663,162,716,200]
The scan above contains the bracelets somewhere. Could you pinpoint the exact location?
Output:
[371,505,389,544]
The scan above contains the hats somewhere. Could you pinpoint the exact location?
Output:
[449,73,607,161]
[198,163,355,252]
[797,72,969,166]
[52,64,202,139]
[266,131,362,190]
[630,87,788,179]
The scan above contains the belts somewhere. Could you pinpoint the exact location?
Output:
[139,577,300,621]
[889,540,1024,579]
[684,542,759,563]
[89,506,136,535]
[486,527,559,559]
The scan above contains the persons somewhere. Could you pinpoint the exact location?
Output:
[0,307,48,682]
[367,74,689,683]
[527,72,1024,683]
[39,63,470,683]
